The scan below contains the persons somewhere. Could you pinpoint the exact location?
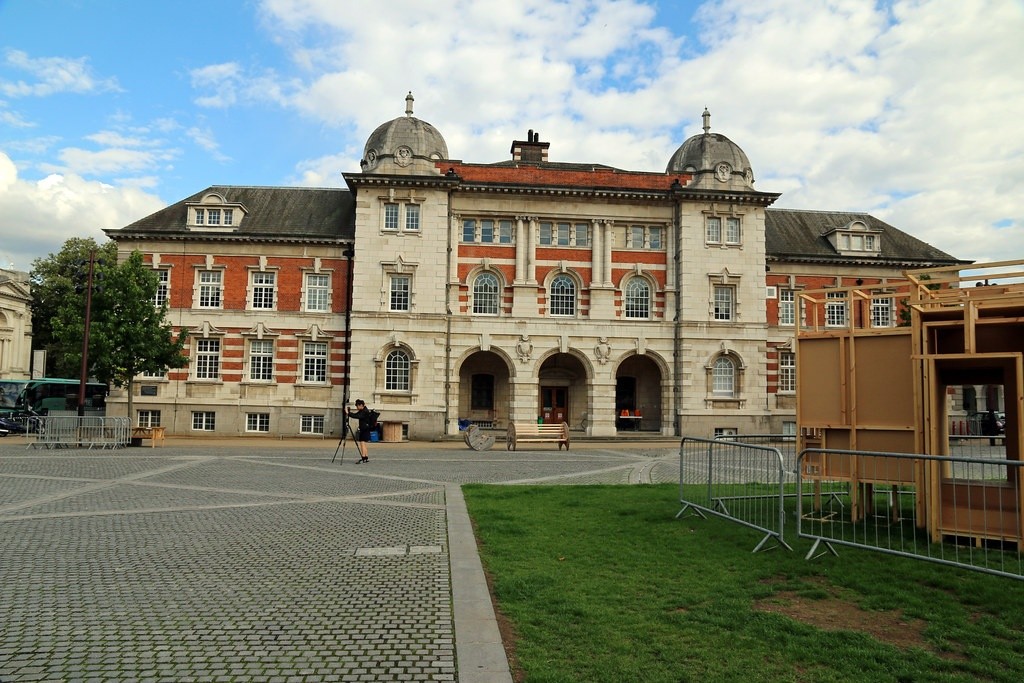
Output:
[346,400,369,464]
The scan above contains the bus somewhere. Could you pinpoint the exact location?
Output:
[0,379,109,433]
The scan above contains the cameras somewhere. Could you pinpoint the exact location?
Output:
[347,407,350,412]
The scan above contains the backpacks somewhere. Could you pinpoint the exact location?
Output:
[369,410,381,431]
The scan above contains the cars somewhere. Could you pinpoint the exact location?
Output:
[972,410,1006,435]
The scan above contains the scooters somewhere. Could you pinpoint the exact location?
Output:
[0,398,44,437]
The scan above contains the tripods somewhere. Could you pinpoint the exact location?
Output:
[332,418,364,465]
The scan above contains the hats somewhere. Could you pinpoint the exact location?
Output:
[355,399,365,406]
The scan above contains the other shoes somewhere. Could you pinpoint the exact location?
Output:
[363,456,369,462]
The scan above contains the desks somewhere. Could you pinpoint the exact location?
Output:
[618,416,643,432]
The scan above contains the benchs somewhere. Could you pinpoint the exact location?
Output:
[507,422,570,451]
[616,420,641,430]
